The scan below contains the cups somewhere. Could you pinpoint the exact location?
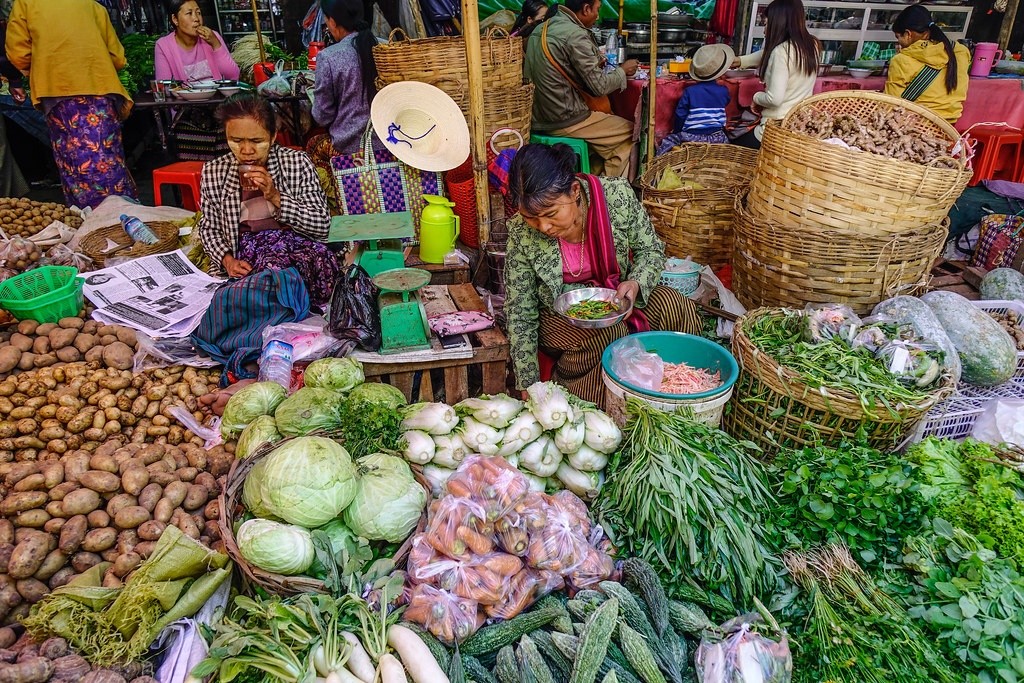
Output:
[254,62,274,85]
[239,163,260,191]
[150,79,166,103]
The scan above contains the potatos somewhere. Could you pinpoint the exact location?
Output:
[1,197,98,322]
[0,317,240,683]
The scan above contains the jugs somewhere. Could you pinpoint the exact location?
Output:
[308,40,324,71]
[419,193,460,264]
[970,42,1003,78]
[958,39,971,52]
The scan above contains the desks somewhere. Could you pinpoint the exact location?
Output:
[607,73,1024,186]
[133,95,309,207]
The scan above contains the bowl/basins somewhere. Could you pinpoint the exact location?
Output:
[590,29,617,43]
[624,22,651,29]
[168,89,182,100]
[657,14,694,24]
[215,80,237,87]
[218,87,241,97]
[627,30,662,43]
[692,29,705,41]
[658,29,690,43]
[818,64,875,79]
[190,81,215,85]
[699,32,717,42]
[193,84,220,90]
[176,89,216,101]
[151,81,182,97]
[553,287,633,329]
[602,331,740,399]
[725,69,755,77]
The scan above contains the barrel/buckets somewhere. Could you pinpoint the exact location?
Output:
[601,368,734,440]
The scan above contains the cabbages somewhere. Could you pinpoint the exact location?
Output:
[397,379,624,501]
[222,355,429,576]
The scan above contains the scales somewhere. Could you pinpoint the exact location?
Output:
[331,211,414,280]
[372,268,432,355]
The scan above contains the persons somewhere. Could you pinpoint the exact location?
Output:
[655,43,737,152]
[190,92,336,383]
[0,0,640,181]
[728,0,821,149]
[505,144,704,412]
[881,4,971,127]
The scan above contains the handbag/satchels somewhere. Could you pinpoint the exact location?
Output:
[257,59,290,98]
[948,183,1024,254]
[330,264,383,352]
[330,117,443,246]
[725,111,760,139]
[579,90,614,114]
[404,455,614,645]
[694,622,792,683]
[132,331,198,372]
[446,127,524,248]
[0,228,97,273]
[972,207,1024,276]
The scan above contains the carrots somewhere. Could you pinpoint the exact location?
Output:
[402,453,620,645]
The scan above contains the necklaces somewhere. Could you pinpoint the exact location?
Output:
[557,189,586,277]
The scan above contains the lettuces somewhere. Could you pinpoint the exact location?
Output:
[903,435,1024,584]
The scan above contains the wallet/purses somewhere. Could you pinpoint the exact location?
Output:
[429,310,496,337]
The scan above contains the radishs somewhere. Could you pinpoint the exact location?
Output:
[307,626,451,683]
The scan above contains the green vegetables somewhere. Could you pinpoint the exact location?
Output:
[115,33,159,94]
[766,521,1024,683]
[254,42,308,70]
[595,314,950,618]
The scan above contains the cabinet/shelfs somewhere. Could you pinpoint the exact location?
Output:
[745,0,974,67]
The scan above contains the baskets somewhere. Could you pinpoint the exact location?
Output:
[0,265,85,323]
[217,428,433,609]
[372,26,535,157]
[901,300,1024,448]
[642,90,974,466]
[79,221,180,268]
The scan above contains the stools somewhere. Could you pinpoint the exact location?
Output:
[955,126,1024,188]
[153,162,206,214]
[530,133,590,176]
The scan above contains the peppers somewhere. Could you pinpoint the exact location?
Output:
[565,297,620,320]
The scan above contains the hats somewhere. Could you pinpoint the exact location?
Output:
[688,43,735,82]
[372,81,471,172]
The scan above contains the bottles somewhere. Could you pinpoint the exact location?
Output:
[258,326,293,393]
[605,29,617,73]
[120,214,159,244]
[617,34,625,66]
